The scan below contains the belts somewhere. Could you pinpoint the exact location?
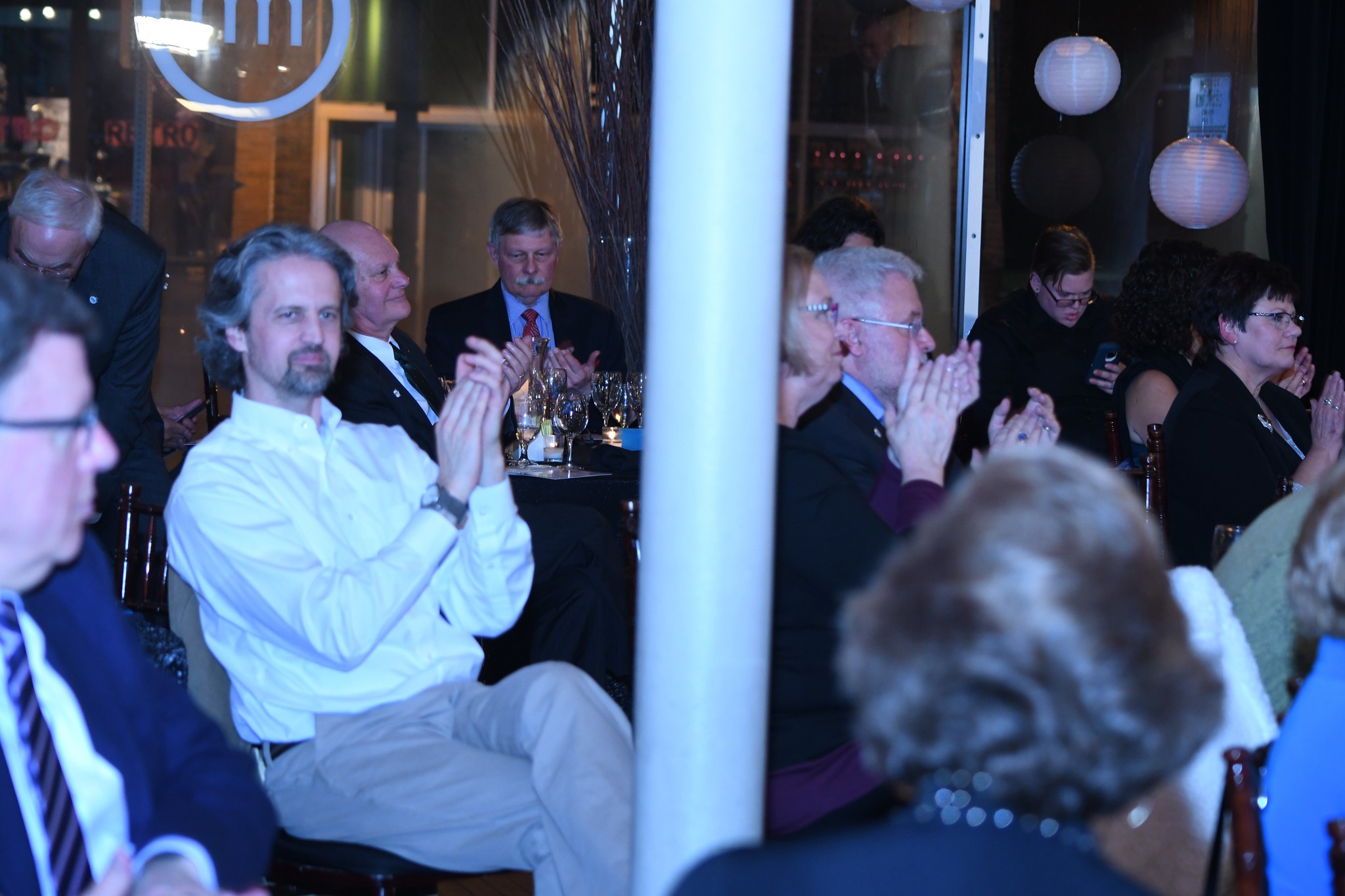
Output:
[259,742,293,767]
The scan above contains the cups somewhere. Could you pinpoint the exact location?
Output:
[438,376,456,397]
[602,427,622,448]
[544,435,565,463]
[1210,524,1247,569]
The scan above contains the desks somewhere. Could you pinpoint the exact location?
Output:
[510,416,640,560]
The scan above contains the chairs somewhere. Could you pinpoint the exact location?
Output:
[109,480,170,626]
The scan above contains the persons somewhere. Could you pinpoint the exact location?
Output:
[794,246,1060,499]
[1112,240,1315,487]
[0,168,278,896]
[1160,251,1345,571]
[670,442,1345,896]
[789,194,884,256]
[765,243,981,827]
[958,225,1125,459]
[319,197,628,464]
[163,222,633,896]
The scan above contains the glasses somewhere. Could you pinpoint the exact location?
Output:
[1040,280,1096,308]
[800,302,839,324]
[1247,312,1304,330]
[5,397,101,447]
[853,318,923,341]
[13,250,89,281]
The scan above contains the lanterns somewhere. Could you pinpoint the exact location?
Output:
[909,0,1248,228]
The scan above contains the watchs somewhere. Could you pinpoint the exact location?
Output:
[420,483,465,524]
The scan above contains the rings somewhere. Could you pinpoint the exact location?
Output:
[1110,381,1115,390]
[1325,397,1333,402]
[1324,399,1332,405]
[1331,404,1339,410]
[1043,424,1049,430]
[1018,432,1027,442]
[518,372,525,378]
[1301,379,1306,385]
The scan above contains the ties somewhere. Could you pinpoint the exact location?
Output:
[391,342,443,418]
[1,603,94,895]
[521,309,541,339]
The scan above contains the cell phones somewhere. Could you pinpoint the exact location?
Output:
[1085,341,1121,385]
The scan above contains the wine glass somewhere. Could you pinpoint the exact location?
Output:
[554,393,589,472]
[609,383,637,429]
[543,368,568,420]
[509,398,544,469]
[591,371,623,449]
[625,371,644,429]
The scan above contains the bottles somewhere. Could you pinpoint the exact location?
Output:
[527,337,550,428]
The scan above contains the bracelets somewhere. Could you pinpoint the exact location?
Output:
[129,834,221,896]
